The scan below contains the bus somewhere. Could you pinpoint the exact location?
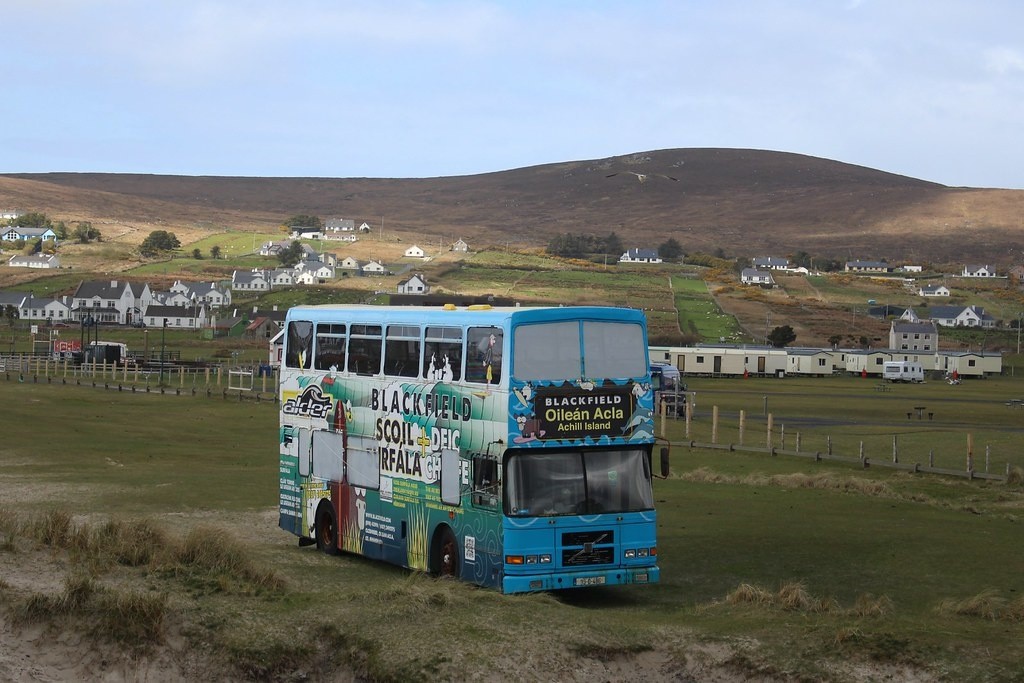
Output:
[277,305,671,596]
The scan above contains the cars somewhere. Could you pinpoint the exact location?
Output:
[133,321,146,328]
[53,323,70,327]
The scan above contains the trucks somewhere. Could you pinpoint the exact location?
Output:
[883,361,926,384]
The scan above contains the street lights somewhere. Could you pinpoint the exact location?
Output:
[12,309,19,358]
[29,290,35,327]
[161,318,168,382]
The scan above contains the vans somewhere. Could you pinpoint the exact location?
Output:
[649,362,688,419]
